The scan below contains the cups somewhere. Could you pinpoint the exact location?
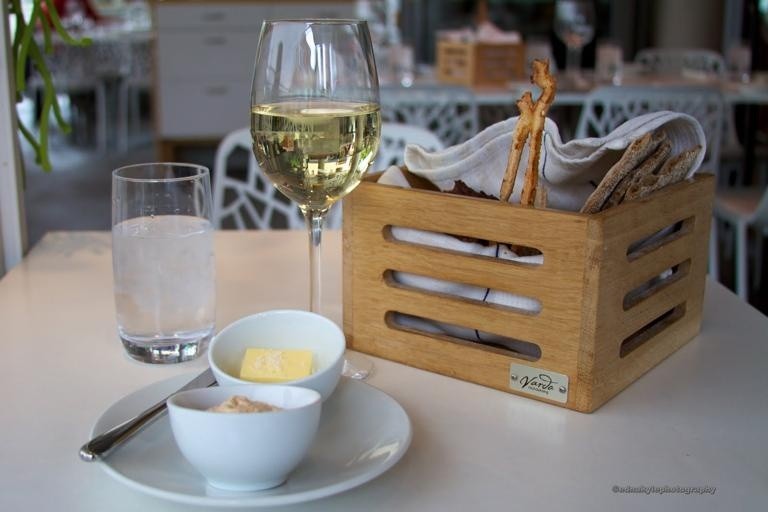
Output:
[110,161,219,366]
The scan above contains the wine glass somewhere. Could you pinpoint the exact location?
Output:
[248,15,375,383]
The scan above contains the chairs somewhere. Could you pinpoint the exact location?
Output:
[211,120,450,233]
[360,46,766,301]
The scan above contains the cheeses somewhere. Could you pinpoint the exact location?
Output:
[237,343,316,386]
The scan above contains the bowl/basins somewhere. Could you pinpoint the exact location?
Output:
[164,382,323,493]
[206,308,348,405]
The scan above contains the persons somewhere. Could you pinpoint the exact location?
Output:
[23,1,114,130]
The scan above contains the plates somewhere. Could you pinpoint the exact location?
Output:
[91,365,416,509]
